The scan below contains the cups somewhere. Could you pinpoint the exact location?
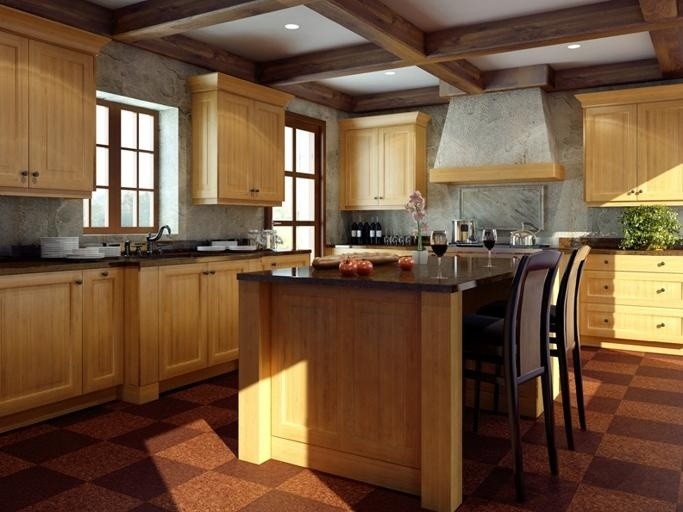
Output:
[482,228,498,268]
[430,229,448,279]
[247,230,278,250]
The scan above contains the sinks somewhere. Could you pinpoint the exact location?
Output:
[160,247,197,253]
[162,253,194,259]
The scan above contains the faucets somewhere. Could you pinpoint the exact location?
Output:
[146,225,171,256]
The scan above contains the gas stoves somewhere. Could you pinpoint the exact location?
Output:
[456,242,550,248]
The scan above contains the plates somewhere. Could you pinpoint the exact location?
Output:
[196,246,257,251]
[39,236,121,259]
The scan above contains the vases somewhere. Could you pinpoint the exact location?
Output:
[411,250,428,264]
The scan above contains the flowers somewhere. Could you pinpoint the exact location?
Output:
[404,190,430,251]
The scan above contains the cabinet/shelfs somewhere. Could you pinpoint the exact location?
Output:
[574,83,683,207]
[337,110,432,210]
[257,252,310,271]
[0,3,114,199]
[0,265,124,435]
[559,253,683,356]
[159,257,257,382]
[188,72,295,207]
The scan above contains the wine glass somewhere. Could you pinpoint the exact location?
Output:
[384,231,418,246]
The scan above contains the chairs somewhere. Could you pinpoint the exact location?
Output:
[462,247,591,505]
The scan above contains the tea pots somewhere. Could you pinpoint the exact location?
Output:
[508,221,538,246]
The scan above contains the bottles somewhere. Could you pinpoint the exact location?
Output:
[351,215,382,245]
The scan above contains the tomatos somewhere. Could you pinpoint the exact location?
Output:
[357,258,374,275]
[338,258,357,276]
[398,257,414,270]
[340,255,355,264]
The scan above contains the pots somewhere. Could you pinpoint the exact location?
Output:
[452,219,478,244]
